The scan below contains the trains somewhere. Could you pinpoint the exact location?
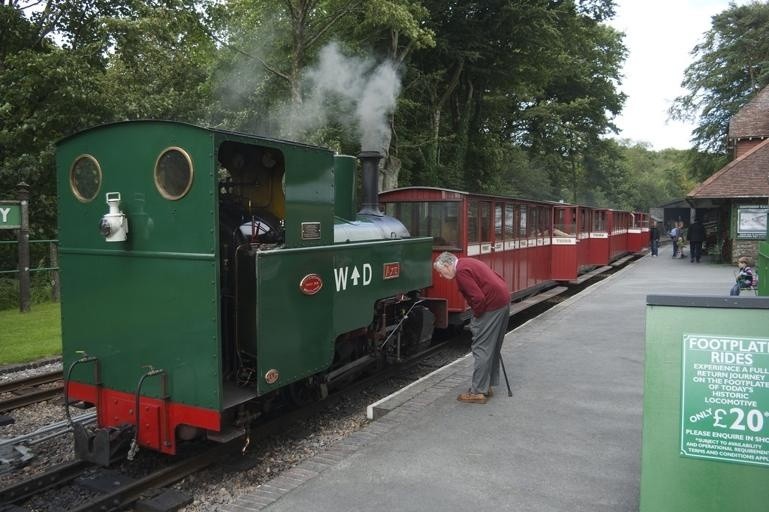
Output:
[54,119,664,470]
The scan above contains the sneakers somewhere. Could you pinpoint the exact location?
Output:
[457,391,487,404]
[469,384,493,397]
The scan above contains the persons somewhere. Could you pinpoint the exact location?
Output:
[649,217,707,263]
[729,255,755,296]
[431,251,511,405]
